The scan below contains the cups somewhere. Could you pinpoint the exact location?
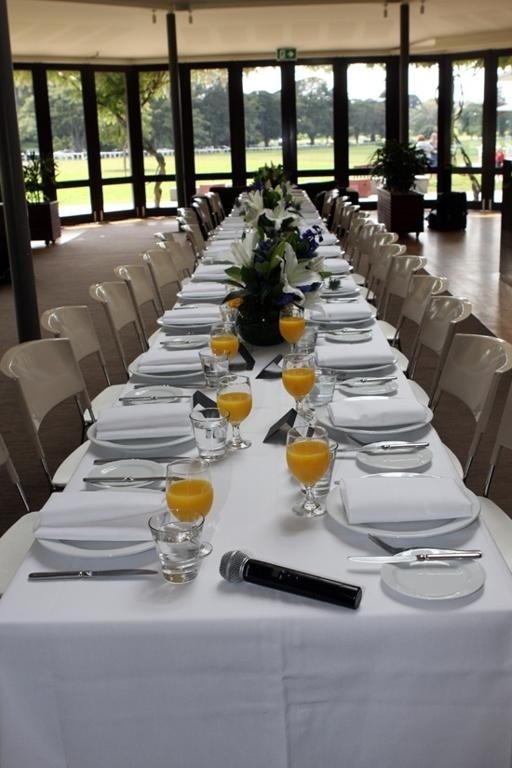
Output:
[220,301,239,325]
[298,353,335,408]
[189,407,230,459]
[301,438,338,495]
[198,347,230,390]
[148,509,205,585]
[229,287,244,293]
[291,315,319,357]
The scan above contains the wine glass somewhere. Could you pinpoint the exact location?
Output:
[282,353,316,416]
[286,425,328,516]
[278,307,306,360]
[227,288,241,307]
[165,457,213,557]
[208,326,238,380]
[212,374,253,450]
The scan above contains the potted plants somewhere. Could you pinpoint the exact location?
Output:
[367,141,434,239]
[22,149,61,247]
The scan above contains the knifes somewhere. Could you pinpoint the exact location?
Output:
[335,442,430,451]
[118,395,193,401]
[346,550,482,567]
[27,569,156,580]
[82,476,185,482]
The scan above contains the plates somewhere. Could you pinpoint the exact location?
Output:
[357,440,435,470]
[124,384,184,405]
[312,233,399,373]
[87,418,196,451]
[84,457,164,489]
[317,395,433,437]
[33,486,168,557]
[326,472,479,537]
[382,549,488,599]
[339,377,398,395]
[128,203,248,378]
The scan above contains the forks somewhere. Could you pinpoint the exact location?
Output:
[366,532,473,556]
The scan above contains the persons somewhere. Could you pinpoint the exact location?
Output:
[428,132,440,167]
[414,134,437,167]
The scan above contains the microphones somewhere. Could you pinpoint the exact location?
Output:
[219,549,362,612]
